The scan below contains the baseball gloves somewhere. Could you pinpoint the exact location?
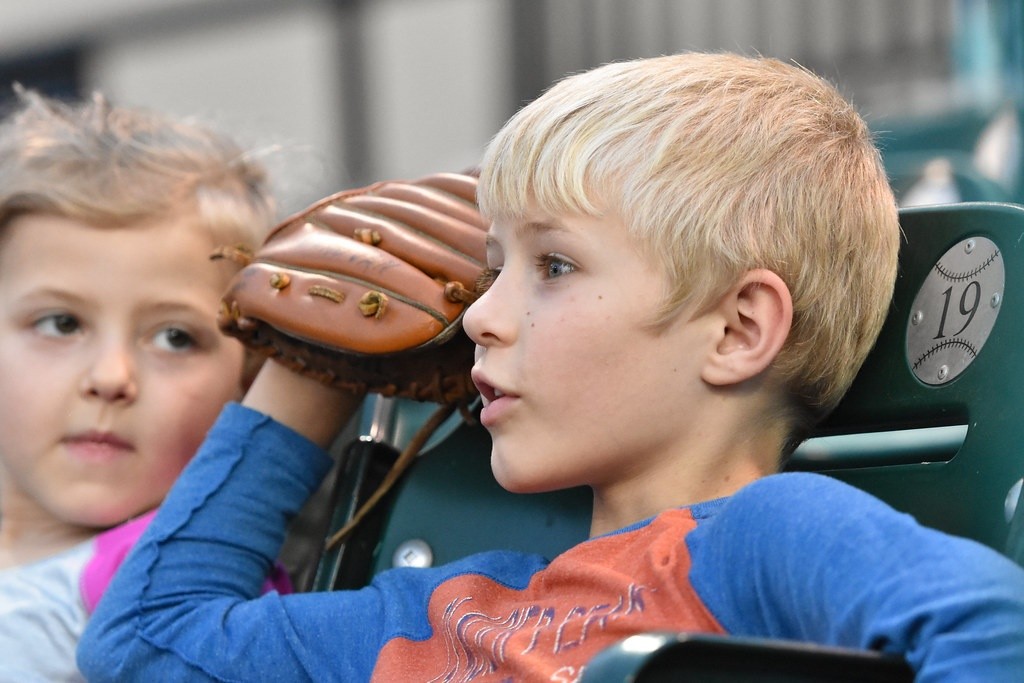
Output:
[214,166,500,408]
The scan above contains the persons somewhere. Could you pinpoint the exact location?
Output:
[1,80,293,683]
[76,41,1024,683]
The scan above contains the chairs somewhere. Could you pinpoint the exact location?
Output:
[311,200,1023,682]
